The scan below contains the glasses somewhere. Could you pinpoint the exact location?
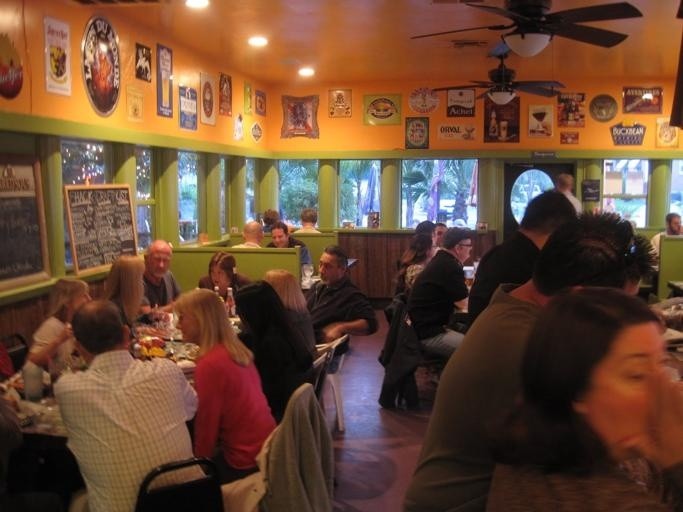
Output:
[460,243,473,247]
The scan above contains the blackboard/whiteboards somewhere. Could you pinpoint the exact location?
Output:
[63,184,138,278]
[0,191,51,291]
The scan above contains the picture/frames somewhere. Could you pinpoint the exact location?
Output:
[81,14,122,118]
[279,94,321,139]
[621,85,665,115]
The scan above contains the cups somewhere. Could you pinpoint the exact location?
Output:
[23,367,43,402]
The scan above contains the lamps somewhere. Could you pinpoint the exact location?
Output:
[500,21,552,58]
[485,87,517,106]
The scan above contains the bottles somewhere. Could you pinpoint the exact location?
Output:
[178,220,196,240]
[226,287,236,317]
[214,287,219,295]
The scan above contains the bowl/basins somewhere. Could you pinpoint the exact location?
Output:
[343,222,351,227]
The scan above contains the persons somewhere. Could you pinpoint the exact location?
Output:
[233,116,243,138]
[135,46,151,81]
[1,205,380,511]
[467,187,579,322]
[330,92,352,117]
[396,208,682,512]
[553,169,585,212]
[160,45,171,73]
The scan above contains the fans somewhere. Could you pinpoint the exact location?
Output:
[432,54,566,97]
[410,1,644,48]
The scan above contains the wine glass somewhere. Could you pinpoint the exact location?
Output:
[129,320,190,360]
